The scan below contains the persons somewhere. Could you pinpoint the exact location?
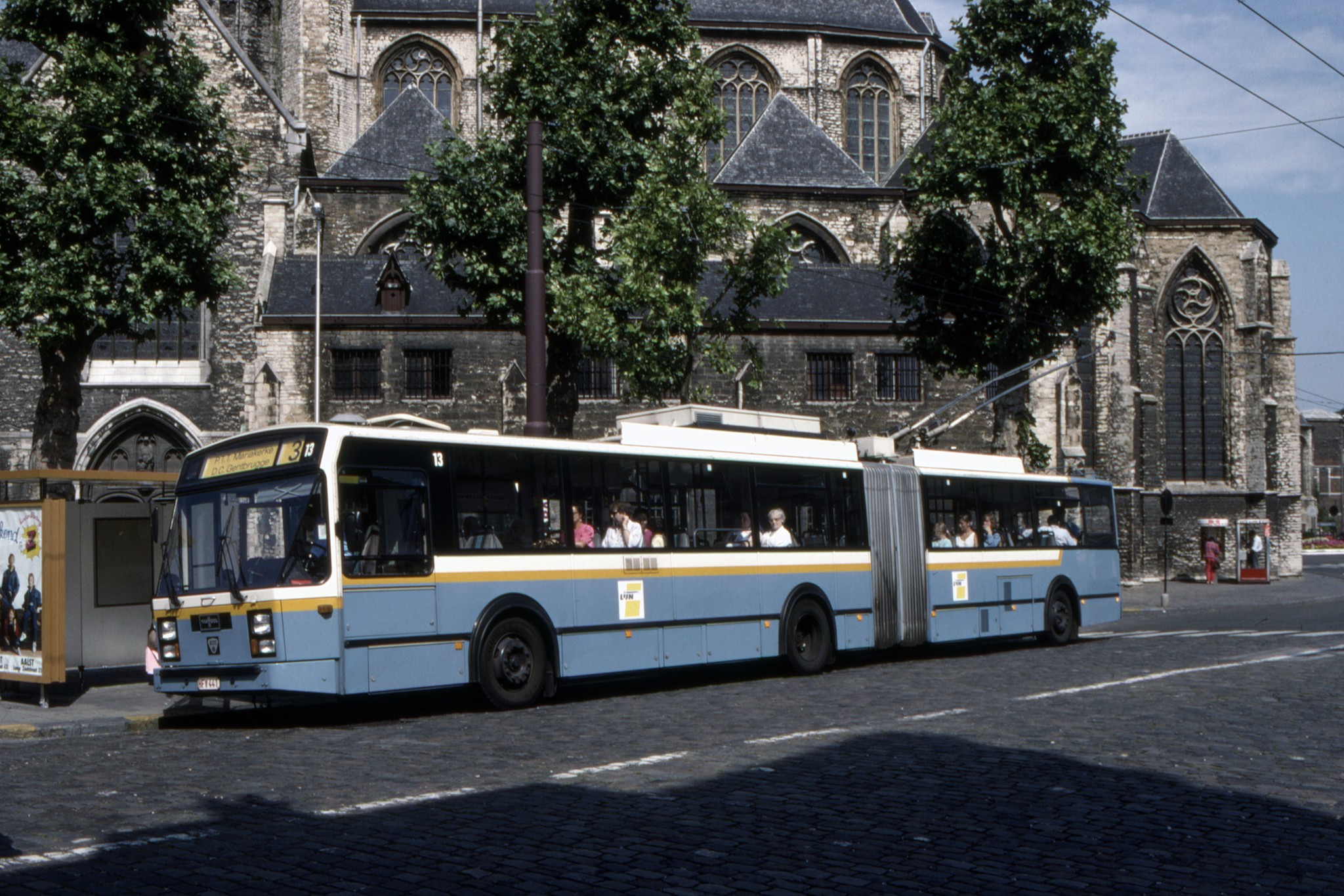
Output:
[0,554,22,656]
[459,516,479,549]
[559,503,594,547]
[1203,537,1221,584]
[955,512,978,547]
[982,513,1002,547]
[715,508,762,547]
[601,500,644,548]
[931,522,953,548]
[1244,530,1263,569]
[19,573,41,652]
[1022,515,1077,546]
[633,508,659,548]
[840,523,862,547]
[761,507,793,547]
[651,518,667,548]
[1061,510,1081,538]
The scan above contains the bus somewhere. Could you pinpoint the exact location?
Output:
[145,334,1121,712]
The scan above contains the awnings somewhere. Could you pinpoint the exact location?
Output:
[1061,446,1087,456]
